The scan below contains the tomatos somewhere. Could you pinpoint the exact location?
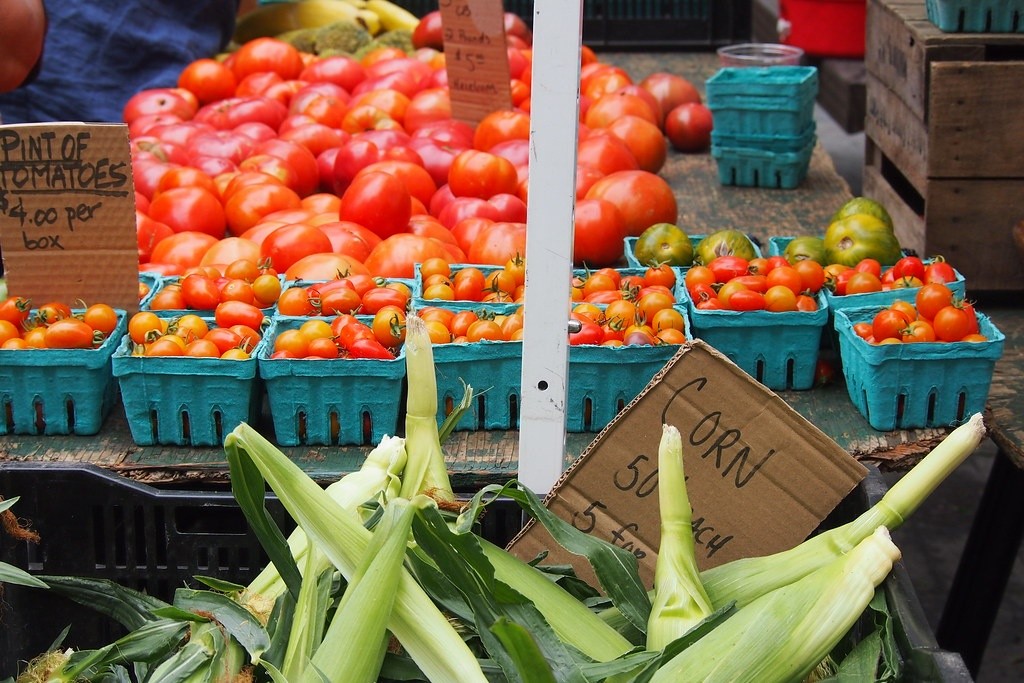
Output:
[784,197,902,268]
[815,355,832,384]
[125,12,711,282]
[0,295,118,428]
[635,221,758,266]
[127,257,282,360]
[570,256,685,347]
[269,267,414,436]
[819,255,988,345]
[414,251,526,345]
[686,256,824,312]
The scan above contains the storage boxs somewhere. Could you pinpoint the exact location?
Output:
[141,272,287,318]
[859,0,1024,295]
[409,305,524,432]
[582,0,752,50]
[623,234,763,274]
[0,462,978,683]
[778,0,867,59]
[925,0,1024,34]
[681,270,830,390]
[257,317,409,446]
[706,65,818,188]
[567,305,692,434]
[273,278,416,318]
[834,306,1006,432]
[767,236,908,270]
[0,270,164,436]
[112,316,268,447]
[572,266,689,308]
[415,262,512,306]
[825,260,967,364]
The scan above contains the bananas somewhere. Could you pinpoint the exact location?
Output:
[216,0,421,57]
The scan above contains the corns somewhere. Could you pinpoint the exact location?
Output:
[170,316,985,683]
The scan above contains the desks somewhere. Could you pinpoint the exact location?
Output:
[1,48,1024,683]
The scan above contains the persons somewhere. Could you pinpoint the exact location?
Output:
[0,0,241,130]
